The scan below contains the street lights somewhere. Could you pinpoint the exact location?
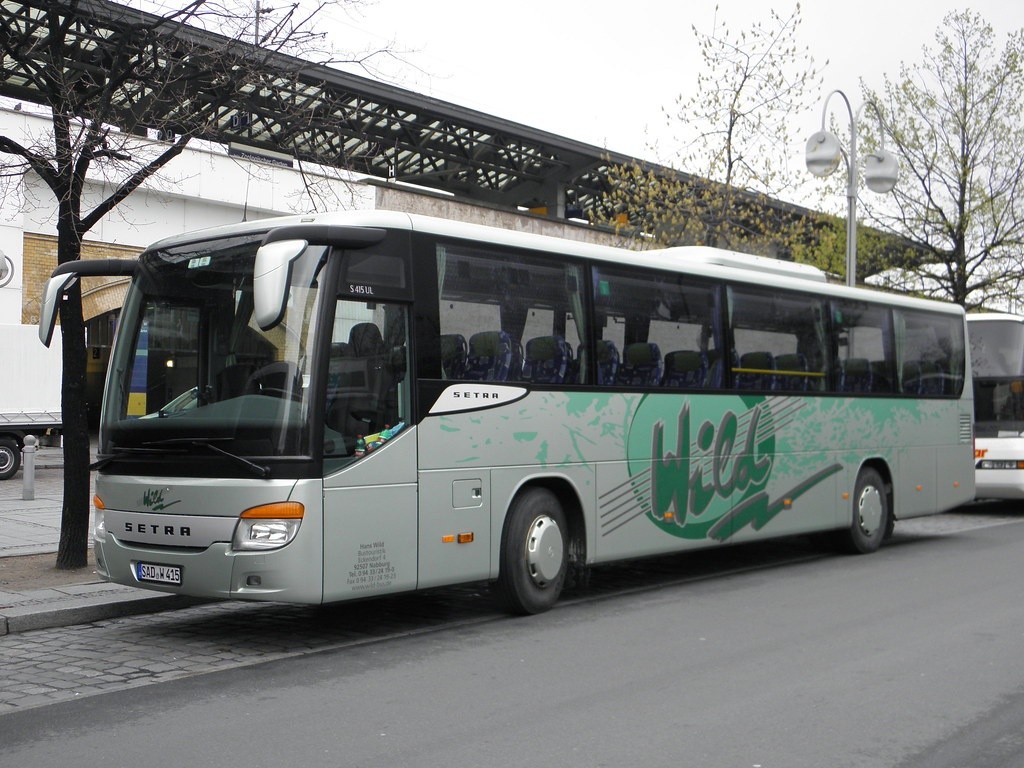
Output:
[802,90,902,286]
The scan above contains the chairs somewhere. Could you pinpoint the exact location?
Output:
[331,324,958,398]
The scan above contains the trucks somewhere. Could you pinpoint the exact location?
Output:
[0,324,88,481]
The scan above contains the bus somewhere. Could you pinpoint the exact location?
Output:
[33,214,977,620]
[953,314,1024,503]
